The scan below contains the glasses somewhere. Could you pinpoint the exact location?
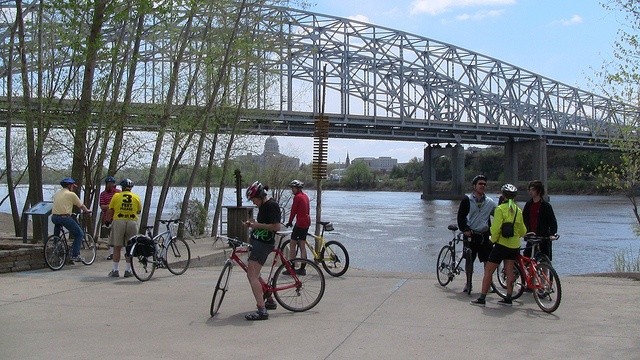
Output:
[474,183,486,185]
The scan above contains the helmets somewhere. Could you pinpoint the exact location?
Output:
[289,180,304,188]
[105,176,116,181]
[120,178,134,187]
[246,181,263,200]
[501,183,517,193]
[60,178,75,184]
[472,174,486,184]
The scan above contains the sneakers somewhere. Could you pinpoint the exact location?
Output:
[265,301,277,310]
[107,254,113,259]
[282,270,291,275]
[245,310,268,320]
[498,299,512,306]
[71,255,85,261]
[109,270,119,277]
[470,298,485,307]
[124,271,133,277]
[296,268,306,275]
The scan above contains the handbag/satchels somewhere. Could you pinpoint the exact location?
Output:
[502,207,518,237]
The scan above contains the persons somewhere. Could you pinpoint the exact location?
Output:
[523,181,556,294]
[282,180,311,275]
[470,184,527,306]
[100,176,122,238]
[240,180,281,320]
[108,178,142,277]
[51,177,90,262]
[457,174,498,294]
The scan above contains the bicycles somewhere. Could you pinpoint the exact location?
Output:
[496,232,562,314]
[437,223,525,300]
[279,221,350,277]
[130,219,191,282]
[43,211,97,271]
[210,231,325,316]
